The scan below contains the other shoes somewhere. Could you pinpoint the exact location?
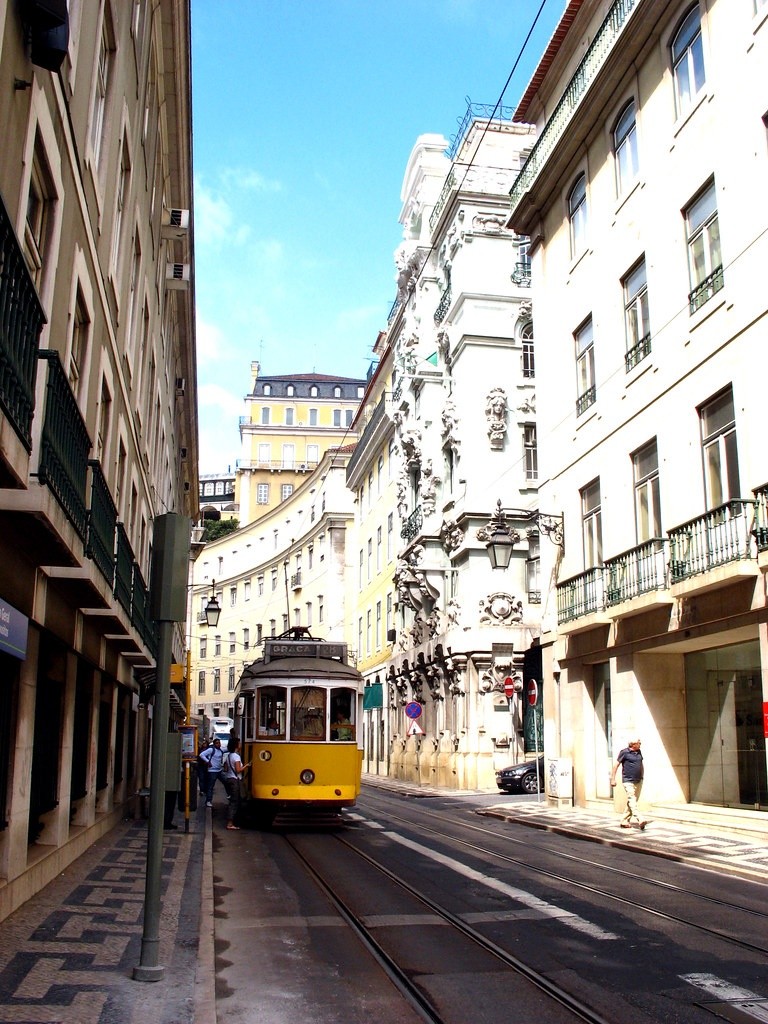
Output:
[200,792,205,796]
[206,801,213,806]
[639,819,648,830]
[620,822,632,828]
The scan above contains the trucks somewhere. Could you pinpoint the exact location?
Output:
[210,716,234,738]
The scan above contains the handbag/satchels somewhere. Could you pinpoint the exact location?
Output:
[238,777,247,800]
[331,721,339,739]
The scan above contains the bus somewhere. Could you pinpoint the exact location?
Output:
[233,625,365,826]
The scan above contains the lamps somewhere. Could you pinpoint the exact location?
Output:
[486,499,567,571]
[187,578,222,628]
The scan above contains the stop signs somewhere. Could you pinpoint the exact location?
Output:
[527,679,537,707]
[504,676,514,699]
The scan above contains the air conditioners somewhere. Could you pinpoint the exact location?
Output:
[176,378,185,396]
[179,446,189,464]
[184,481,191,495]
[166,261,193,291]
[161,209,189,241]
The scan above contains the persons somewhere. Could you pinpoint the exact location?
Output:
[199,739,229,806]
[224,739,252,830]
[164,791,177,829]
[199,741,209,796]
[333,711,351,740]
[610,737,647,829]
[268,719,278,735]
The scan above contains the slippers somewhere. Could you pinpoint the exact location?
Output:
[226,825,241,830]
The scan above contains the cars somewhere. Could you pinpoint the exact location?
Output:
[495,752,544,795]
[211,732,231,752]
